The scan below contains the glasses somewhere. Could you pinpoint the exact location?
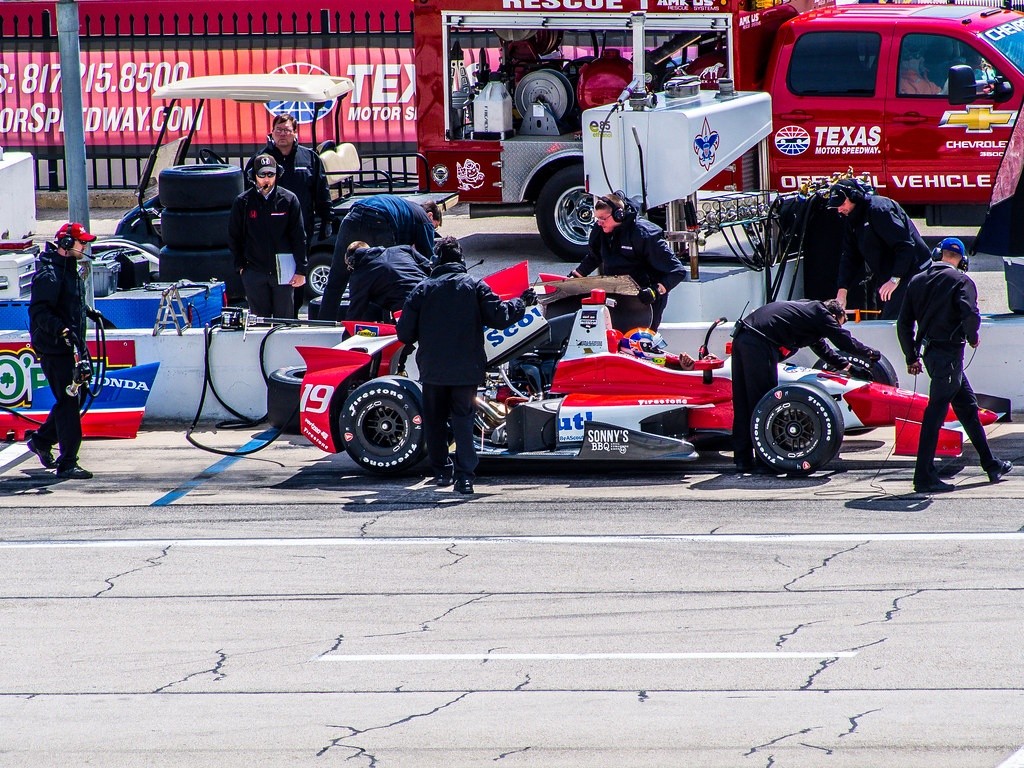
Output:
[432,217,438,231]
[275,127,292,134]
[77,239,88,245]
[591,214,612,222]
[256,172,275,178]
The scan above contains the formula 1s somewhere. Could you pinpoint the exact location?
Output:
[267,273,1011,480]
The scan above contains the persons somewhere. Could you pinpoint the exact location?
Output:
[227,155,308,327]
[898,40,942,95]
[567,194,686,333]
[827,179,933,320]
[317,194,442,322]
[243,113,335,245]
[896,238,1012,493]
[396,235,539,496]
[27,222,97,479]
[345,241,433,325]
[731,300,882,478]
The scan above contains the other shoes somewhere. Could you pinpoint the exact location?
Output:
[737,458,755,473]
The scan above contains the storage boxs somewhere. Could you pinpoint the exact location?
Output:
[92,260,122,297]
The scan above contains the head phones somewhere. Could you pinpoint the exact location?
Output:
[247,162,284,179]
[829,184,864,204]
[931,242,969,273]
[601,196,625,223]
[428,245,439,270]
[346,248,356,267]
[59,222,75,250]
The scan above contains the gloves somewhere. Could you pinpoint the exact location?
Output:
[522,287,539,306]
[864,348,881,363]
[638,283,660,305]
[87,306,103,322]
[317,223,333,241]
[61,329,79,349]
[848,365,874,382]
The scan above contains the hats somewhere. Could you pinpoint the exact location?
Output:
[940,238,965,257]
[825,179,856,210]
[255,153,277,174]
[56,224,97,242]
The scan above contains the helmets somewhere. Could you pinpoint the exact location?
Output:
[618,327,668,369]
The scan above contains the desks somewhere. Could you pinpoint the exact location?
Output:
[0,282,225,328]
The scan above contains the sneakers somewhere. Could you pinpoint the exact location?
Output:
[914,478,954,493]
[436,471,454,485]
[986,461,1013,484]
[454,479,473,494]
[27,438,57,468]
[57,465,93,479]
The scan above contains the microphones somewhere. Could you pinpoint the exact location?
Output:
[248,177,268,189]
[66,246,96,260]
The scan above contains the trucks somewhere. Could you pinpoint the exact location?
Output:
[410,0,1024,260]
